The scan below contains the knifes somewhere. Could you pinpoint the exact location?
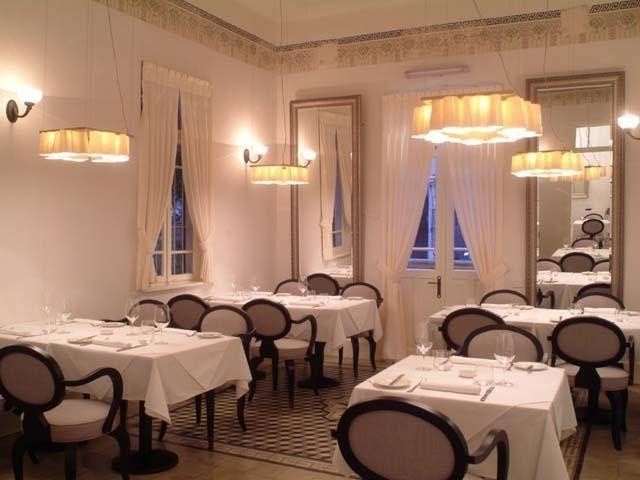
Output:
[480,385,494,404]
[388,372,405,385]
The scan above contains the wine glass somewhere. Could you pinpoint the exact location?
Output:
[39,276,335,345]
[411,319,518,386]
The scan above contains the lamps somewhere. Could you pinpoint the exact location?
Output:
[409,1,543,147]
[38,0,134,165]
[249,0,308,186]
[298,149,316,166]
[6,89,43,122]
[510,1,585,178]
[548,124,606,184]
[244,144,268,163]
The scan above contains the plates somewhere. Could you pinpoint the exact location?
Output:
[514,360,548,372]
[347,296,366,302]
[373,376,411,390]
[495,305,640,327]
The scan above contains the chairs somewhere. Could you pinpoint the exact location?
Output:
[534,211,612,310]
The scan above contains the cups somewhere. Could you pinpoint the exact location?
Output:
[569,301,586,315]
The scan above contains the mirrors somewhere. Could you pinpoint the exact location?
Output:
[290,93,363,296]
[524,69,623,310]
